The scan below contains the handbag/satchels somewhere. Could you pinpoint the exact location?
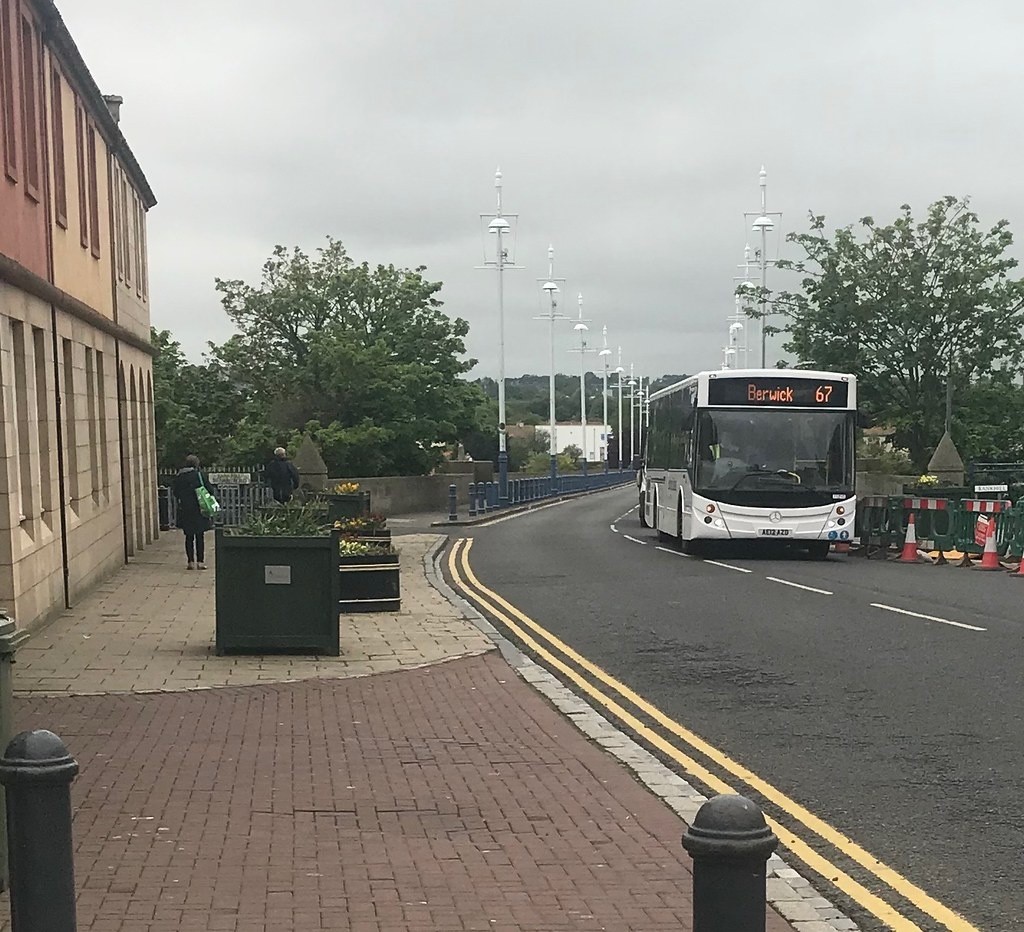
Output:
[195,472,220,518]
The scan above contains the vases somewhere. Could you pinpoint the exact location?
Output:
[354,529,392,548]
[903,484,976,537]
[338,553,401,614]
[306,490,370,523]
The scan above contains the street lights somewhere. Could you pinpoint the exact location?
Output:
[742,162,783,368]
[534,244,566,497]
[736,242,760,367]
[721,282,748,368]
[597,324,614,490]
[613,345,626,485]
[571,293,594,492]
[627,363,652,482]
[472,163,518,511]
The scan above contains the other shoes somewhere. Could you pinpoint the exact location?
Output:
[187,561,194,570]
[197,562,207,569]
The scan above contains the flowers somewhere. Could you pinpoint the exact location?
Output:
[906,473,959,489]
[331,515,402,557]
[326,482,361,494]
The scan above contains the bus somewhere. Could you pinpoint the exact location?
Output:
[633,369,857,560]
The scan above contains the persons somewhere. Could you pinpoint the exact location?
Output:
[173,454,215,570]
[709,412,846,485]
[264,446,299,504]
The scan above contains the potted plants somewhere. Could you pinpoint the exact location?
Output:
[214,493,342,657]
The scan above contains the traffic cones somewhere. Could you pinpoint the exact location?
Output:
[894,512,924,564]
[1009,546,1024,577]
[969,516,1006,571]
